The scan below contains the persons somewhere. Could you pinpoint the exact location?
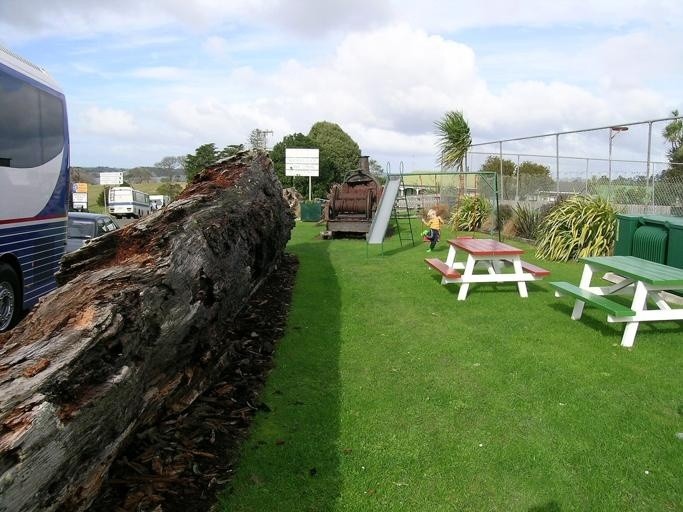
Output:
[150,200,157,212]
[422,208,444,252]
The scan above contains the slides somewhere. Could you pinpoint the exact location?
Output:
[366,177,401,257]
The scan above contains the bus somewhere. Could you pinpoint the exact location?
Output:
[0,47,70,333]
[108,187,170,219]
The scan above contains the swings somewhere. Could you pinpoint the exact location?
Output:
[419,175,440,242]
[456,174,478,239]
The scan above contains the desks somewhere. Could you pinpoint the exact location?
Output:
[571,255,683,348]
[439,239,529,301]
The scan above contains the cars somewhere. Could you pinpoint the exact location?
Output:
[66,212,120,253]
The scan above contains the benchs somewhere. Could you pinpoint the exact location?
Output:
[424,257,462,285]
[548,278,636,322]
[603,271,682,305]
[500,256,551,284]
